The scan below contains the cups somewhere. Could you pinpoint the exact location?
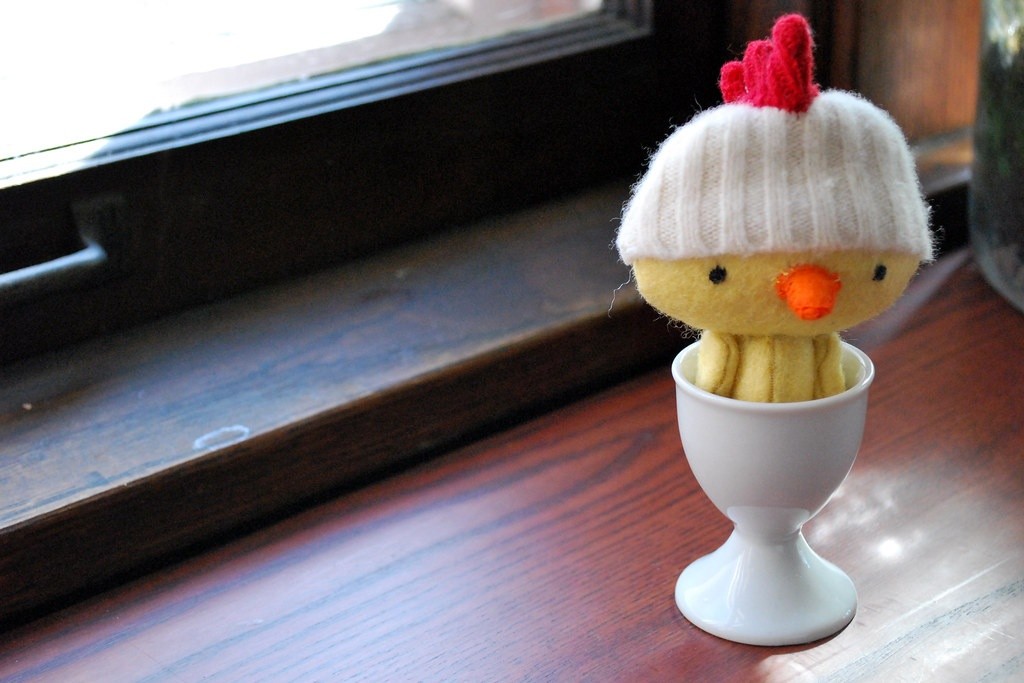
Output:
[963,0,1024,311]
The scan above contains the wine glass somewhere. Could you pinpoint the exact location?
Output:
[671,339,875,647]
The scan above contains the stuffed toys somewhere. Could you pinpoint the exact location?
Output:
[614,15,934,405]
